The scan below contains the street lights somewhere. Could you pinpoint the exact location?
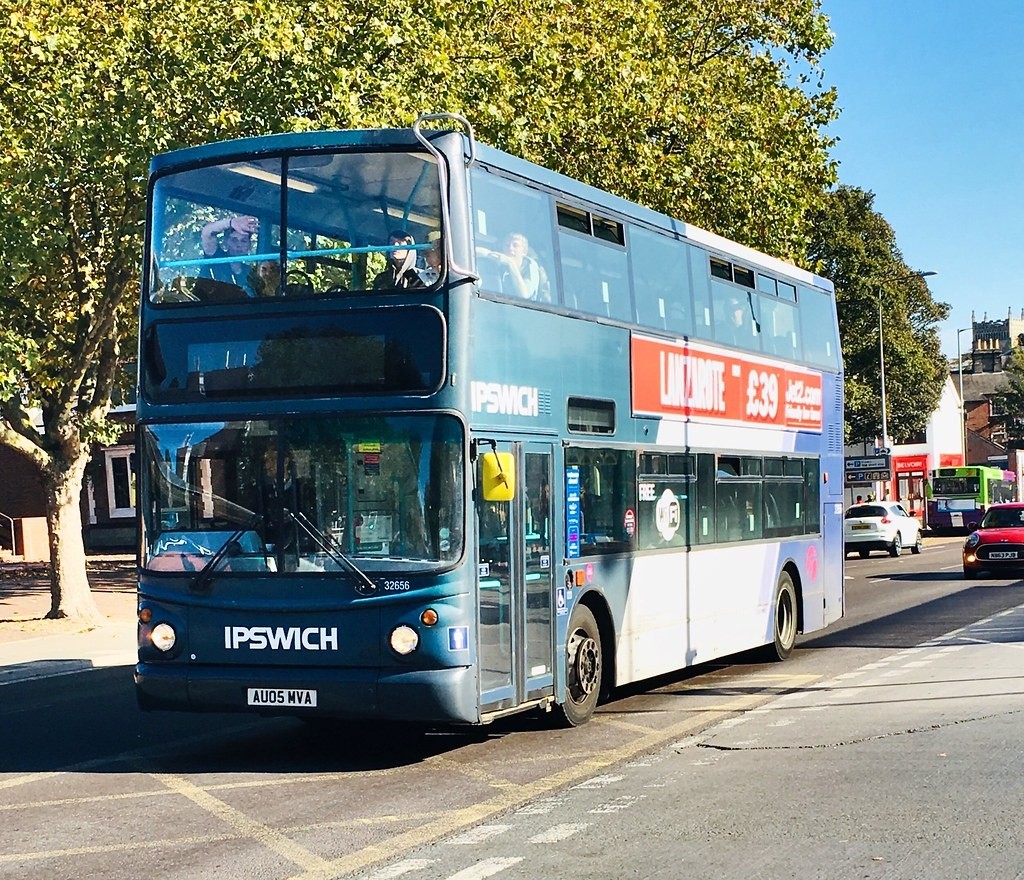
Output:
[875,271,938,448]
[957,322,1004,467]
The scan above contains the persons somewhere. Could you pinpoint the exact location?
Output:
[187,215,281,301]
[372,230,539,301]
[723,298,753,349]
[856,494,874,504]
[245,445,327,552]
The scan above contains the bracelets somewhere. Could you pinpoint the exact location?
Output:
[230,217,235,231]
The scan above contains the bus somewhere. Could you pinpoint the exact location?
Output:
[106,111,848,736]
[927,466,1017,535]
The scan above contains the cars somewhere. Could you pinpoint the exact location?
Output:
[844,501,924,558]
[963,502,1024,575]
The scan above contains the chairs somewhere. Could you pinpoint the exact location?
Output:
[277,261,838,370]
